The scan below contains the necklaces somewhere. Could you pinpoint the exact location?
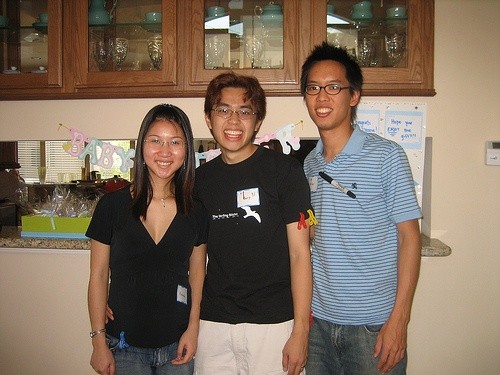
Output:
[161,198,165,207]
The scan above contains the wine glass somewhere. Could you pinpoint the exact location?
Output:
[146,37,162,70]
[93,37,128,72]
[207,37,264,68]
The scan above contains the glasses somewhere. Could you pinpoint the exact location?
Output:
[142,138,187,148]
[304,84,353,95]
[210,107,259,120]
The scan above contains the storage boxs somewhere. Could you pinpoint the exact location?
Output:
[21,214,93,240]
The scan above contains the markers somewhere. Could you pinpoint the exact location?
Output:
[319,171,356,199]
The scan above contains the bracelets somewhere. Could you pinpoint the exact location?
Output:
[90,328,105,337]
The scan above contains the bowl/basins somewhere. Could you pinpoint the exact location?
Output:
[0,15,9,28]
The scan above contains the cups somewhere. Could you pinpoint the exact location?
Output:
[329,32,406,67]
[386,7,407,31]
[39,13,48,23]
[40,67,44,70]
[144,12,161,22]
[260,59,271,68]
[207,6,224,18]
[11,67,17,70]
[38,167,77,185]
[348,1,373,29]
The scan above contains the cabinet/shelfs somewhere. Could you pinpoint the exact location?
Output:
[0,0,437,102]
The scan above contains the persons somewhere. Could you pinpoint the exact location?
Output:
[193,73,315,375]
[300,41,423,375]
[85,104,209,375]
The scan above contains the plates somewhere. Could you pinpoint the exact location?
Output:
[205,18,240,23]
[32,70,48,73]
[140,22,162,32]
[4,71,20,74]
[32,23,48,31]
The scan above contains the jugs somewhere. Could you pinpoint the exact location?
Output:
[89,0,118,24]
[253,1,283,27]
